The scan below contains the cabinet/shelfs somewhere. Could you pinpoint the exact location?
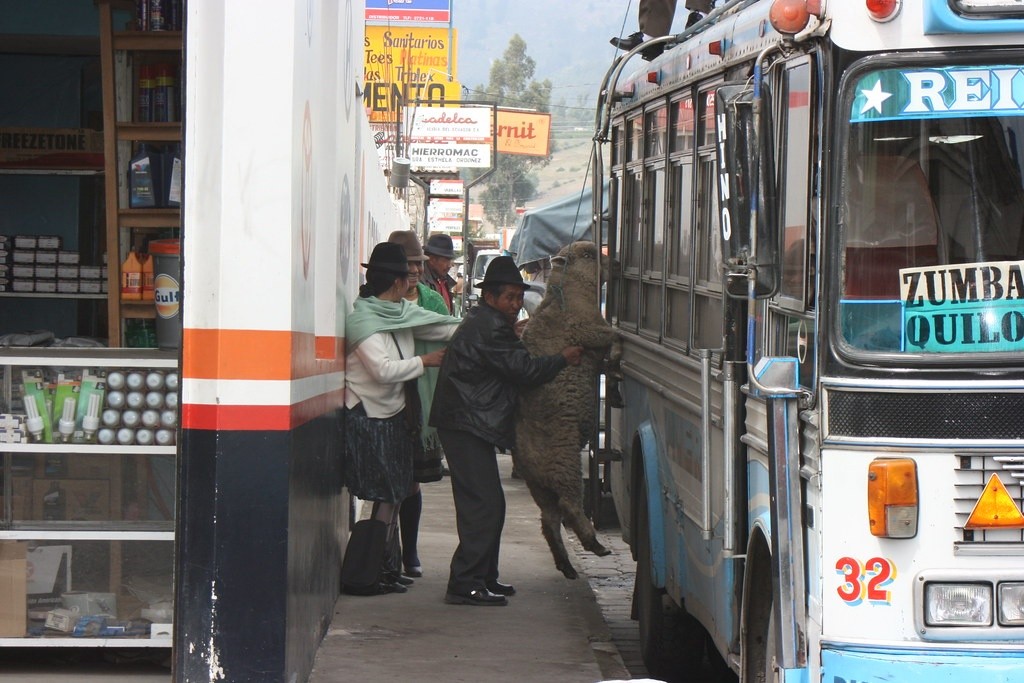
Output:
[0,34,105,349]
[103,0,186,627]
[0,347,180,648]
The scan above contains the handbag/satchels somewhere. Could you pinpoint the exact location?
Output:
[341,518,387,596]
[411,430,444,482]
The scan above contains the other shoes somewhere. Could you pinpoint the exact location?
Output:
[381,570,414,585]
[446,587,506,606]
[406,565,422,577]
[489,582,513,594]
[380,581,407,592]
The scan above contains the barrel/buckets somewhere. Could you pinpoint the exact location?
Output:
[147,239,180,348]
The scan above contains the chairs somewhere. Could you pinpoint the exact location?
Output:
[847,153,950,300]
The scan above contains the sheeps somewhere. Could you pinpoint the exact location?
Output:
[512,240,620,581]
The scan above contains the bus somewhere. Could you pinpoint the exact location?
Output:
[593,0,1024,683]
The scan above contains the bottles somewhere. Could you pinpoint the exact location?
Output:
[120,250,154,300]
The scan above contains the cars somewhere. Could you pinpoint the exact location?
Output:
[449,237,610,528]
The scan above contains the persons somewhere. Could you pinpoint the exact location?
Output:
[429,255,586,606]
[610,0,715,62]
[388,230,452,577]
[339,243,465,596]
[419,234,458,476]
[452,272,464,318]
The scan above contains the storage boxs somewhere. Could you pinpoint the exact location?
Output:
[0,126,102,169]
[0,474,116,638]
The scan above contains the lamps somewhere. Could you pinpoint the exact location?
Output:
[390,157,410,188]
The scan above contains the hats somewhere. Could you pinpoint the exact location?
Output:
[360,242,410,273]
[473,256,530,290]
[388,230,430,260]
[422,234,457,258]
